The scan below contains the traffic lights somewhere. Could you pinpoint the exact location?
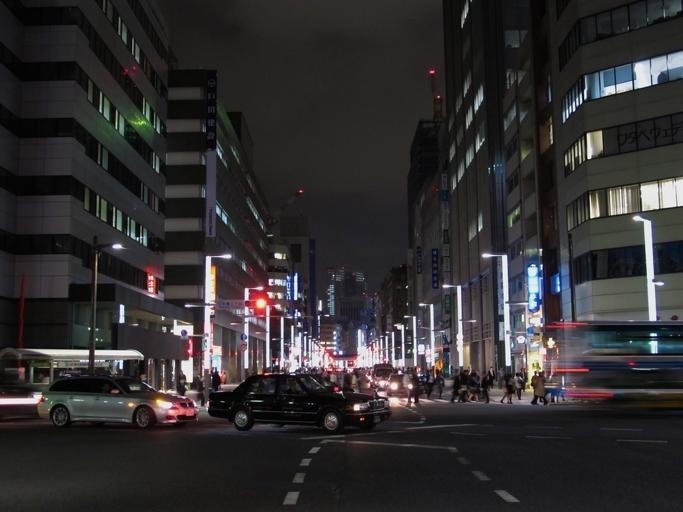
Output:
[252,298,270,310]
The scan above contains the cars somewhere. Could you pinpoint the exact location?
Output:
[37,374,197,428]
[371,364,424,398]
[208,371,391,432]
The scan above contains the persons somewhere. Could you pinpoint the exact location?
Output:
[501,368,562,405]
[298,365,373,394]
[390,366,496,404]
[177,367,226,406]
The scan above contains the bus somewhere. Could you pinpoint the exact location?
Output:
[542,320,683,410]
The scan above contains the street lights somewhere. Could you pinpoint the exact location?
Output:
[243,286,263,372]
[481,253,511,375]
[203,253,231,400]
[419,303,434,366]
[403,315,418,368]
[370,324,405,367]
[442,284,463,373]
[265,304,322,369]
[634,216,660,353]
[89,243,123,376]
